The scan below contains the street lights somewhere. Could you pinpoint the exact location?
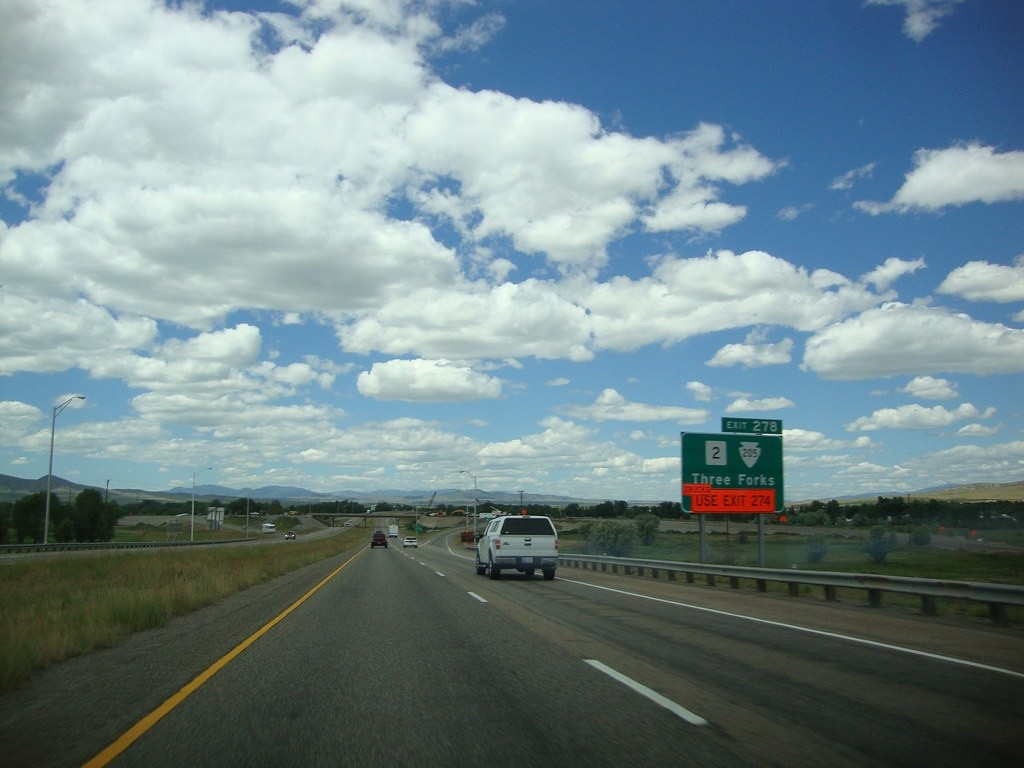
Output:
[42,395,87,544]
[190,466,213,542]
[459,470,477,547]
[456,489,469,533]
[246,488,260,538]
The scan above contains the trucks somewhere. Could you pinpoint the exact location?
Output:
[388,525,399,537]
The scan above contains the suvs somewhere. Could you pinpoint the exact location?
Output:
[284,531,296,540]
[470,515,560,580]
[370,533,387,548]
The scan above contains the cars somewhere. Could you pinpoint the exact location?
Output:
[402,536,418,548]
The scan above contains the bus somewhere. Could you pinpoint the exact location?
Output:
[262,523,276,533]
